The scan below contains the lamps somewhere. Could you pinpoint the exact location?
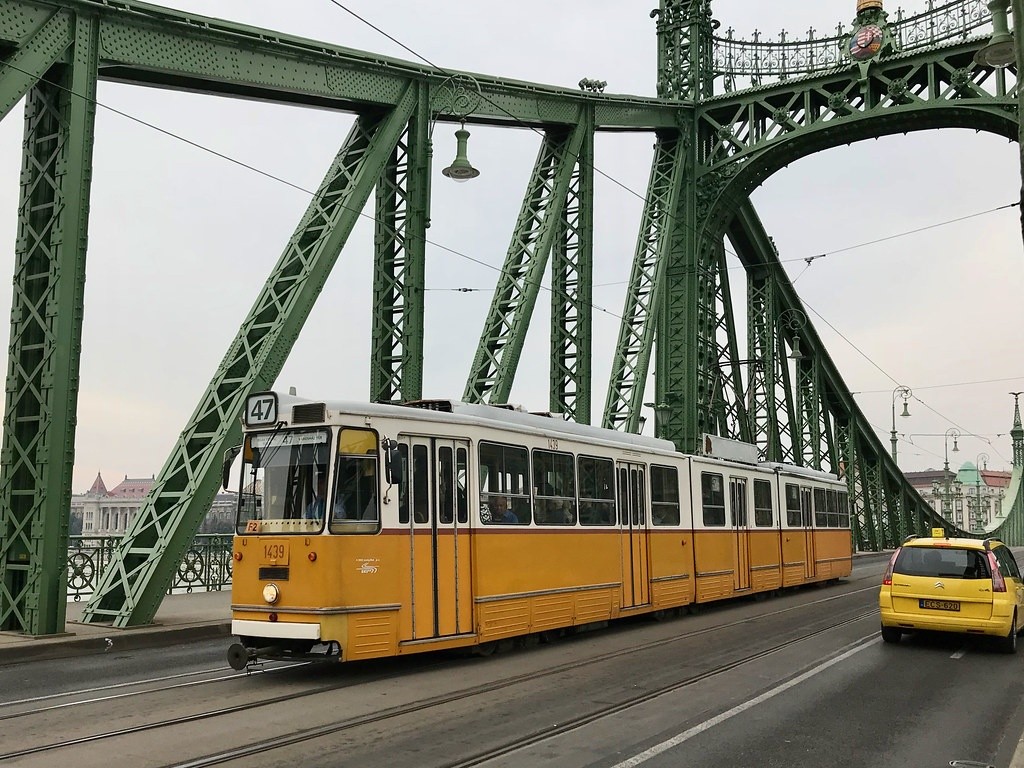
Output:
[426,72,483,232]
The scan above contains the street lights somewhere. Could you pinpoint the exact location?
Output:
[889,383,913,464]
[943,426,961,525]
[974,452,991,534]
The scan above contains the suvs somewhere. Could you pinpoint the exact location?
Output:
[878,534,1024,654]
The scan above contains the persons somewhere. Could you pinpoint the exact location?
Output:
[299,475,347,518]
[488,495,519,523]
[533,480,611,526]
[653,505,678,524]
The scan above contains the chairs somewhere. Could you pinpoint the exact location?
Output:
[924,550,942,574]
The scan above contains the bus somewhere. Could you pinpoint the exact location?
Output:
[220,384,862,682]
